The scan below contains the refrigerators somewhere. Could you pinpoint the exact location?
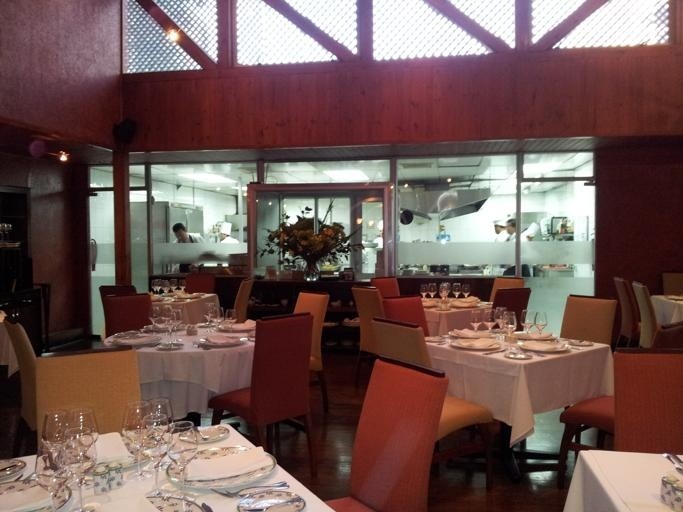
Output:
[130,202,204,293]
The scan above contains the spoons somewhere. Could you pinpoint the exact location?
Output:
[663,454,683,474]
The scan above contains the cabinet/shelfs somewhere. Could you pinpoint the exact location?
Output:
[0,183,45,400]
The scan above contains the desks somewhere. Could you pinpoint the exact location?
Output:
[651,294,681,327]
[105,322,255,425]
[426,332,614,474]
[578,451,683,512]
[3,425,337,512]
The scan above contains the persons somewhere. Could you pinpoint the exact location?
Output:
[172,223,205,273]
[218,221,240,245]
[503,218,530,277]
[494,220,510,242]
[525,222,539,242]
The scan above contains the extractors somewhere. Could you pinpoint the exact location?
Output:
[398,185,491,219]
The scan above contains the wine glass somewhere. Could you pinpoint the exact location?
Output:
[150,278,186,296]
[147,303,237,349]
[39,396,198,512]
[467,304,546,351]
[419,282,471,306]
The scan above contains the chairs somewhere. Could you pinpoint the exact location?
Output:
[613,346,682,454]
[34,344,145,456]
[613,277,640,352]
[209,313,317,477]
[93,272,333,321]
[560,292,621,349]
[294,292,330,388]
[334,272,535,346]
[634,282,681,353]
[100,293,155,335]
[370,317,495,511]
[558,322,682,481]
[326,354,449,512]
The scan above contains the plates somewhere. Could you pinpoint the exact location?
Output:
[148,423,304,512]
[107,319,259,353]
[0,456,71,510]
[449,329,593,360]
[417,296,481,312]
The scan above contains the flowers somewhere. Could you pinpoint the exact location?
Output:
[260,206,369,259]
